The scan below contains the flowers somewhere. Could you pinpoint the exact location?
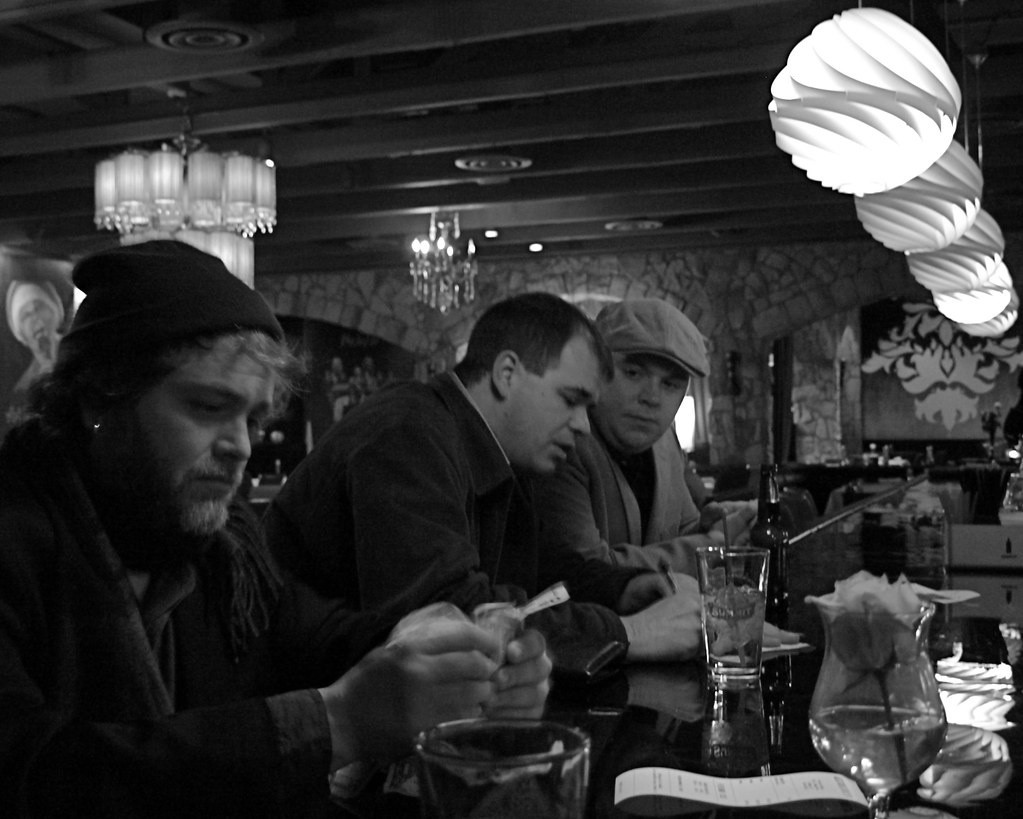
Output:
[802,568,981,781]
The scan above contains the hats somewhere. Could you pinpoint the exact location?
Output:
[57,239,287,368]
[598,297,709,380]
[6,277,64,344]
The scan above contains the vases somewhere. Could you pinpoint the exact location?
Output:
[805,601,948,819]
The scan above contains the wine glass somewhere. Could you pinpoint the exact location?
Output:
[807,592,950,819]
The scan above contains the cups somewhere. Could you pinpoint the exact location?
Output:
[695,544,771,683]
[412,716,591,819]
[700,684,770,778]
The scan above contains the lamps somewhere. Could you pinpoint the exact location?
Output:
[90,76,278,286]
[404,202,479,312]
[767,0,1018,339]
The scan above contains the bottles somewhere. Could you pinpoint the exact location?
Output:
[760,653,793,754]
[750,463,796,620]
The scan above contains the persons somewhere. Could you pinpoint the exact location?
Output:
[7,274,62,394]
[0,239,553,819]
[550,669,712,723]
[1003,367,1023,463]
[264,291,760,710]
